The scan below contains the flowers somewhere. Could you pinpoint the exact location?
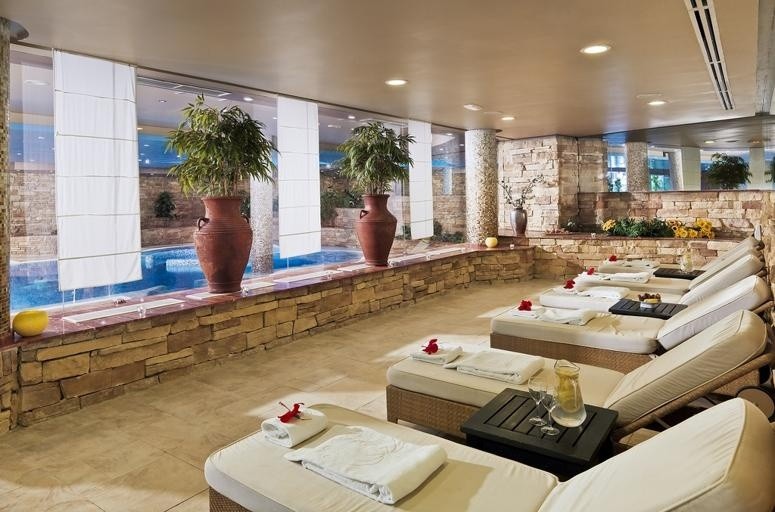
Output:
[667,218,715,239]
[601,218,618,232]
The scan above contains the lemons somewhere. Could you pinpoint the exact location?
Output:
[556,366,582,413]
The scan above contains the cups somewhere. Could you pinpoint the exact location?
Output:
[510,243,514,249]
[426,252,430,259]
[327,272,332,280]
[138,306,145,317]
[591,233,595,237]
[551,359,587,428]
[462,248,466,253]
[243,286,249,296]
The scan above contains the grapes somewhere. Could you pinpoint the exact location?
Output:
[638,293,660,301]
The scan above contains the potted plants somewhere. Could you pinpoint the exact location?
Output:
[499,176,544,235]
[163,94,280,293]
[330,120,417,266]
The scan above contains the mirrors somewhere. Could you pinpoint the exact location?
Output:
[578,115,775,191]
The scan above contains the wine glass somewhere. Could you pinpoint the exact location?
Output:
[527,377,560,436]
